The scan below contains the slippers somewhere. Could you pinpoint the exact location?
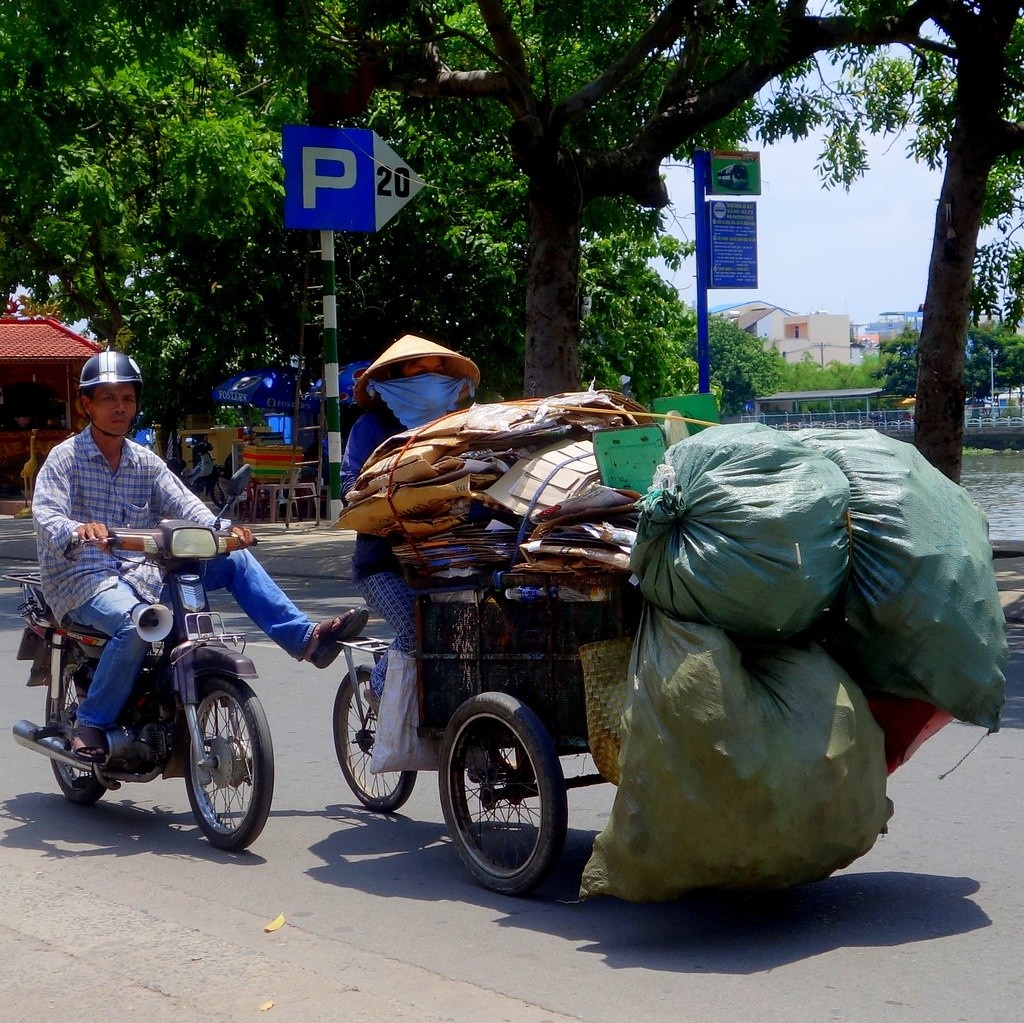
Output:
[70,727,106,763]
[364,689,380,718]
[311,608,369,669]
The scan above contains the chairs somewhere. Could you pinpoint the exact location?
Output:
[264,467,301,520]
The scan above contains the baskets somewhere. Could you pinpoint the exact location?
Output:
[578,637,635,786]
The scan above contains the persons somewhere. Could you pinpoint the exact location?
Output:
[339,333,480,713]
[30,352,369,765]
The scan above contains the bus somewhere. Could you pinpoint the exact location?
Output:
[715,163,750,189]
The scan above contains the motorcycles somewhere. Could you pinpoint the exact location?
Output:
[0,461,275,852]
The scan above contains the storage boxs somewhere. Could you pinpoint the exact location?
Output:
[243,442,303,479]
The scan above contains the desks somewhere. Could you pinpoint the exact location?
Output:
[252,483,318,523]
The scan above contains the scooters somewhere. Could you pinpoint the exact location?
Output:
[160,435,236,510]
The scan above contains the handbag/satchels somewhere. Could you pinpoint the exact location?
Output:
[370,652,482,774]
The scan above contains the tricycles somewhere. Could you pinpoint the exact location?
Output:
[333,566,649,897]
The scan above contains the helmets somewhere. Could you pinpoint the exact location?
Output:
[79,351,144,390]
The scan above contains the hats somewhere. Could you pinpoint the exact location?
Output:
[354,336,481,411]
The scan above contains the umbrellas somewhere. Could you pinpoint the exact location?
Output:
[212,362,371,444]
